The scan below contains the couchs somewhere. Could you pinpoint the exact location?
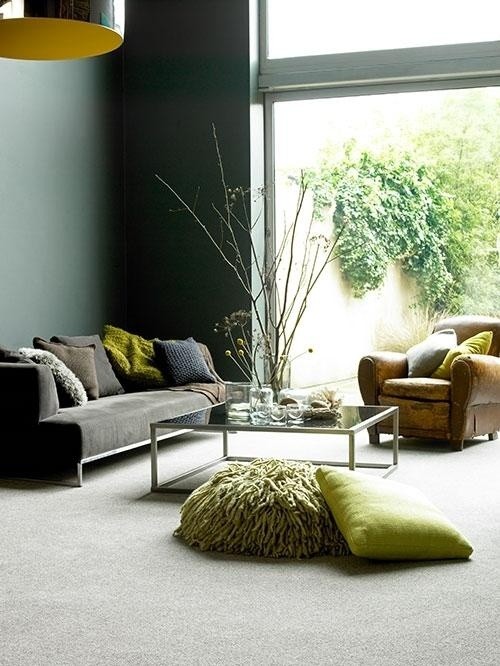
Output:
[0,342,214,487]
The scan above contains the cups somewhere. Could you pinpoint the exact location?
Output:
[270,402,305,424]
[225,382,252,424]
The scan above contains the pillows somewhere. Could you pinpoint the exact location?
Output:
[0,324,217,408]
[315,466,473,564]
[405,328,494,380]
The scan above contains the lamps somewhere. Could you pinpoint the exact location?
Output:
[0,0,126,62]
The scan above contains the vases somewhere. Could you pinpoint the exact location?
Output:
[262,353,291,426]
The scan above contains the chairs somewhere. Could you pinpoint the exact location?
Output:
[357,313,500,451]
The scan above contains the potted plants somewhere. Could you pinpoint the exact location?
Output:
[153,119,386,402]
